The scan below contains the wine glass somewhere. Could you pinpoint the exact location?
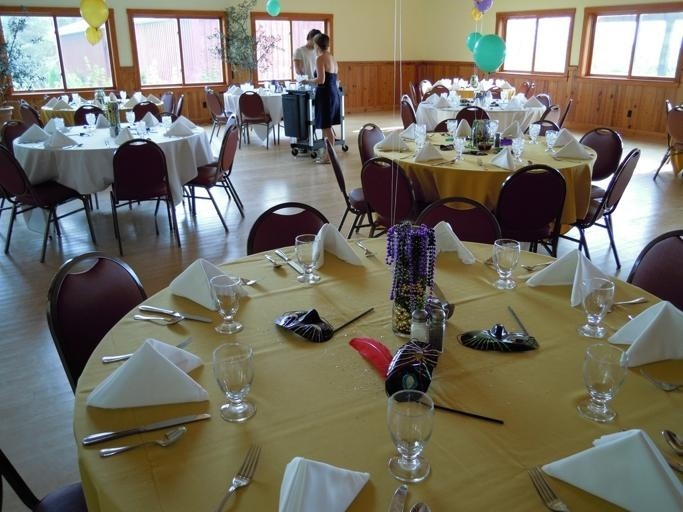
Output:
[211,341,258,424]
[544,130,556,153]
[54,117,64,133]
[446,119,456,138]
[294,234,321,284]
[510,137,524,163]
[575,342,628,423]
[485,120,498,141]
[134,121,146,139]
[527,124,540,145]
[383,390,435,483]
[490,238,521,291]
[208,274,244,336]
[125,111,135,127]
[162,116,171,133]
[413,123,426,157]
[85,113,95,128]
[453,137,465,160]
[575,278,614,339]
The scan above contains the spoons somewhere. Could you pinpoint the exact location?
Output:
[132,314,185,325]
[521,260,553,272]
[660,427,682,457]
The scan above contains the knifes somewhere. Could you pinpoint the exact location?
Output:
[79,412,210,448]
[385,484,410,512]
[275,249,304,274]
[139,304,211,323]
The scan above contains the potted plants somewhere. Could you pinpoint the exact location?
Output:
[0,19,45,124]
[472,118,498,151]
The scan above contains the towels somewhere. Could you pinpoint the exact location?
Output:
[167,115,197,130]
[18,123,51,143]
[553,139,591,158]
[527,250,611,307]
[415,143,443,162]
[375,129,407,151]
[86,339,209,409]
[95,112,110,128]
[44,130,80,147]
[163,122,194,137]
[113,127,137,143]
[313,221,361,270]
[490,147,514,170]
[454,119,472,137]
[169,257,246,312]
[501,121,521,137]
[541,428,681,511]
[141,111,158,127]
[430,217,477,266]
[278,457,371,512]
[550,128,576,147]
[608,300,681,367]
[399,122,417,140]
[43,118,69,135]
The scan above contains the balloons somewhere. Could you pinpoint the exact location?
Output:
[83,27,103,48]
[472,1,492,13]
[472,33,507,79]
[264,0,281,16]
[464,33,481,53]
[77,1,109,32]
[471,8,483,23]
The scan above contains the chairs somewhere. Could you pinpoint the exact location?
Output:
[578,128,623,181]
[323,137,379,238]
[0,120,29,156]
[523,120,562,137]
[360,157,417,237]
[109,138,182,256]
[247,203,330,255]
[19,91,185,122]
[188,159,231,203]
[413,197,501,245]
[434,119,466,133]
[0,449,90,511]
[400,77,574,130]
[358,123,384,166]
[624,227,681,311]
[182,125,246,233]
[653,99,683,181]
[493,165,566,259]
[239,91,275,150]
[45,252,148,395]
[568,148,641,266]
[204,86,245,144]
[0,145,97,263]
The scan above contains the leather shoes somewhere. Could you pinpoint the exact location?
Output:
[314,156,332,164]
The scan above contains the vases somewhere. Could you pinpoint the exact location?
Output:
[107,101,120,137]
[389,228,432,338]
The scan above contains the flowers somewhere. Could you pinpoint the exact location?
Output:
[382,219,436,310]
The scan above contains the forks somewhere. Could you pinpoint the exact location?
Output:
[101,336,193,363]
[639,367,682,393]
[98,426,187,458]
[215,446,263,512]
[526,465,569,512]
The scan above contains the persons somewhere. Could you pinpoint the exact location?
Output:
[294,29,322,155]
[299,34,340,163]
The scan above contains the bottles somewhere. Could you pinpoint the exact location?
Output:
[288,82,295,90]
[428,309,445,353]
[410,308,429,345]
